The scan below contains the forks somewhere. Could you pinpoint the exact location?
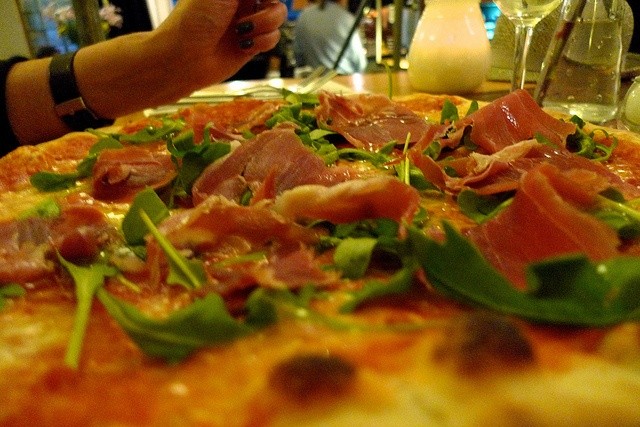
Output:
[210,63,326,90]
[189,68,338,100]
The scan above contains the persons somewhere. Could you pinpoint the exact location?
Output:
[293,0,369,79]
[0,0,289,154]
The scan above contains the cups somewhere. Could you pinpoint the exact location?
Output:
[533,0,624,126]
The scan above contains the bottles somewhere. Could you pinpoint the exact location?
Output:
[406,0,491,93]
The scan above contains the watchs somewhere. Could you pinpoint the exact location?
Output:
[47,48,117,134]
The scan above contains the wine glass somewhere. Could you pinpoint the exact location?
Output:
[494,0,564,105]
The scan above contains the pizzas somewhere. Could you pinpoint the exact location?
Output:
[1,87,640,427]
